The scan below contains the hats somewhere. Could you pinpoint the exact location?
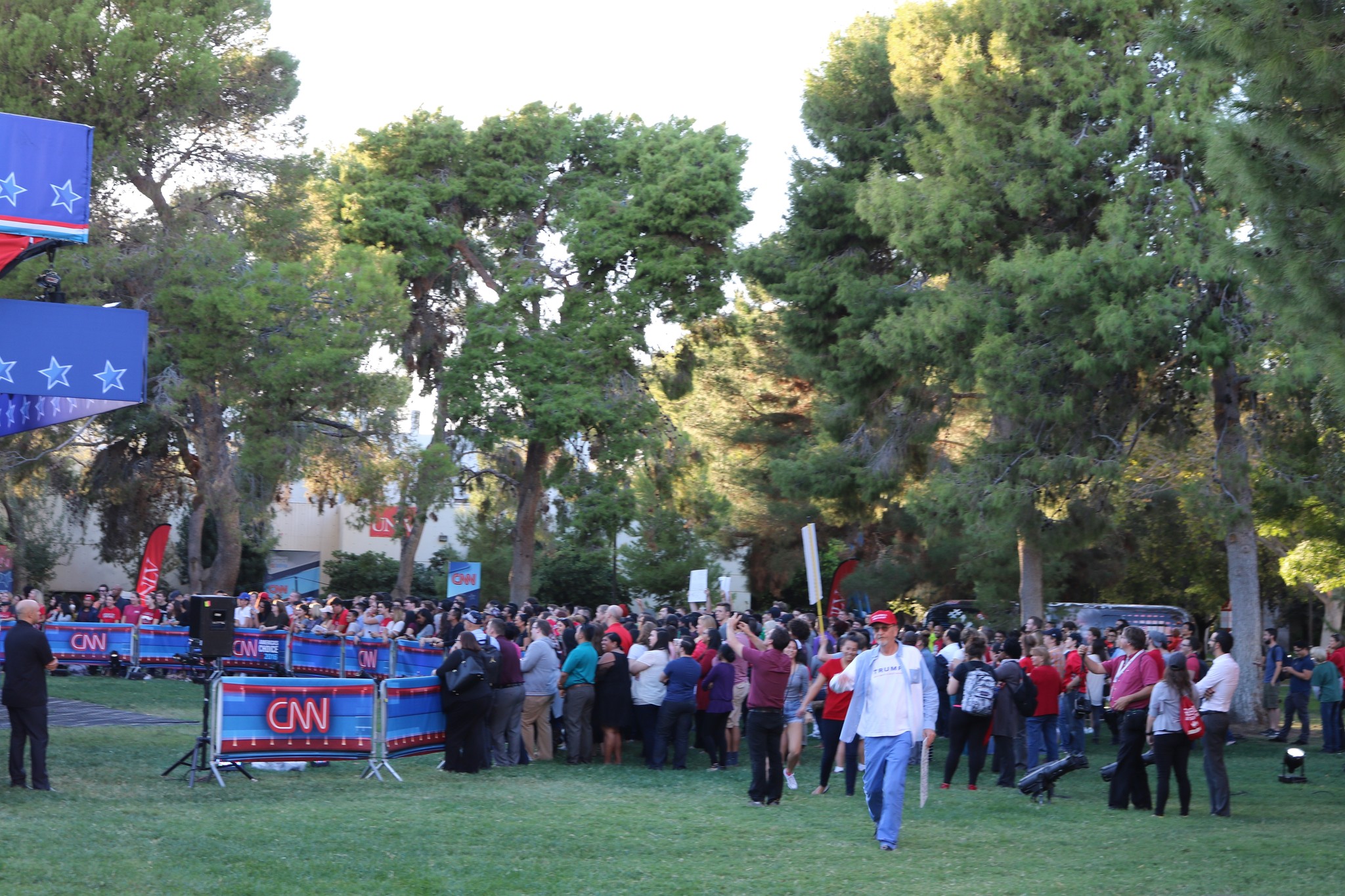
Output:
[1168,652,1186,671]
[1041,627,1063,641]
[868,610,897,627]
[764,606,794,623]
[1148,630,1167,647]
[1067,633,1082,641]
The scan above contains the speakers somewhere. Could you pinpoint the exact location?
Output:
[187,593,236,659]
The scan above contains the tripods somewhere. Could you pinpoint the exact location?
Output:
[160,657,263,791]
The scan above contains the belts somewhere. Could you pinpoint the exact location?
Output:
[491,683,523,689]
[570,683,594,688]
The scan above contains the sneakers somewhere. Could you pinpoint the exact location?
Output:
[143,673,187,681]
[707,751,867,807]
[967,785,976,791]
[940,783,949,790]
[1224,727,1344,755]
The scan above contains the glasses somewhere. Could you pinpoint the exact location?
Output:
[1208,639,1216,642]
[1181,644,1191,646]
[872,625,897,632]
[1293,648,1305,652]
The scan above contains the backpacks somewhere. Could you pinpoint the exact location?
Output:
[480,635,509,686]
[1186,652,1209,681]
[1002,660,1039,718]
[1272,645,1293,681]
[962,661,996,717]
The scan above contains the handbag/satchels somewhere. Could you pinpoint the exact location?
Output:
[1075,696,1092,715]
[445,649,484,695]
[1178,687,1204,739]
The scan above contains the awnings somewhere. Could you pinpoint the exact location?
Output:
[1,296,150,439]
[0,111,94,282]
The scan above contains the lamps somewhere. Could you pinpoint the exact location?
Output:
[1019,750,1090,804]
[356,668,380,699]
[1276,747,1309,783]
[1099,748,1158,782]
[109,650,123,679]
[270,661,294,677]
[171,653,203,684]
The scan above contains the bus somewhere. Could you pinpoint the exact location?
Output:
[922,601,1201,669]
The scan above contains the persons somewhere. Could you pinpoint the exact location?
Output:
[830,610,939,852]
[0,573,1345,818]
